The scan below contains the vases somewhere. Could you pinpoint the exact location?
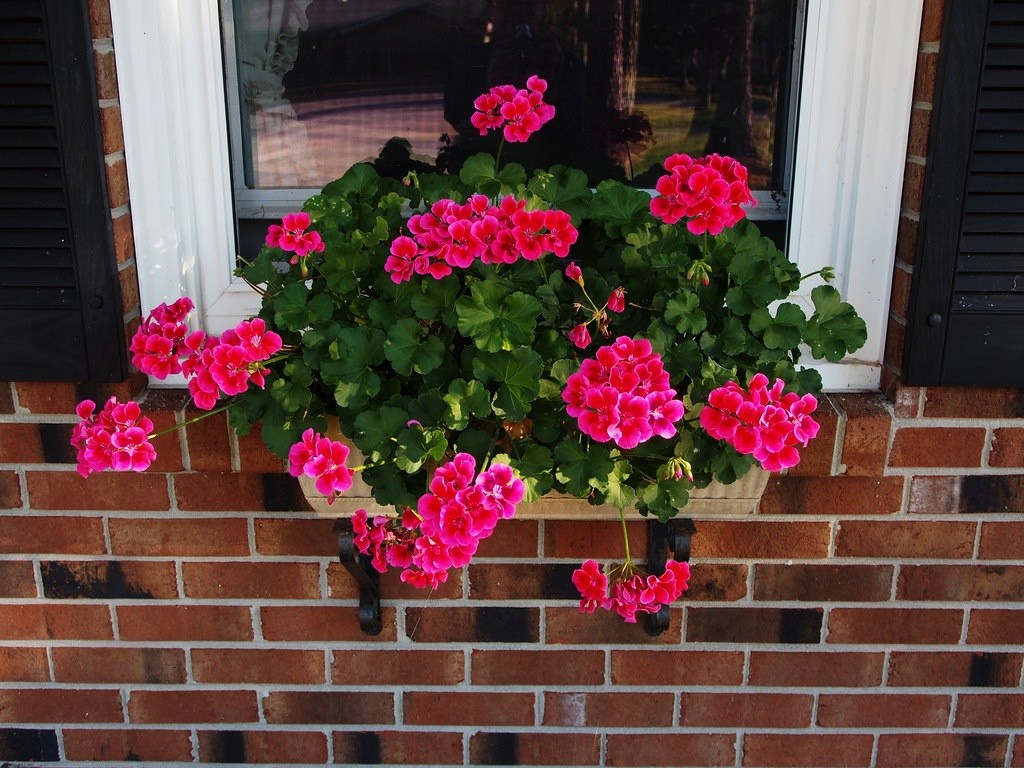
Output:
[294,414,769,523]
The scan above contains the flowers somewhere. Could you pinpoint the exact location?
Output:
[68,72,869,624]
